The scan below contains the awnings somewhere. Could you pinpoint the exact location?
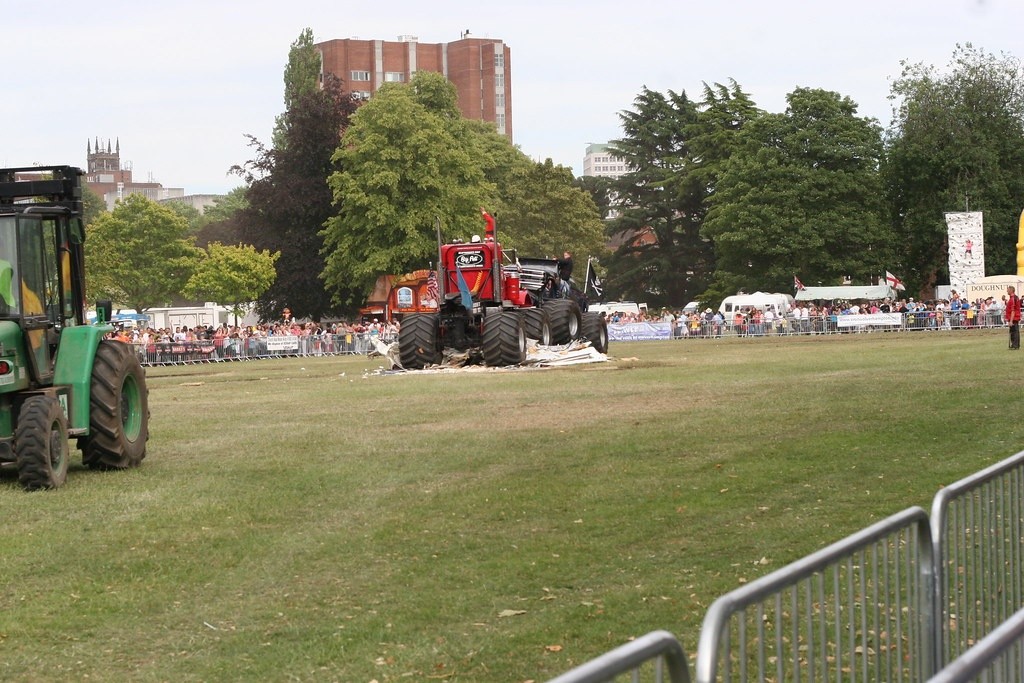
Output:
[795,285,898,301]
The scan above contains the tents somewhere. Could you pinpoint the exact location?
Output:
[976,275,1024,284]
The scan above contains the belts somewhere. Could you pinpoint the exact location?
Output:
[486,232,493,234]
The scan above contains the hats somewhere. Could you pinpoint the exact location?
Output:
[662,307,666,310]
[706,308,712,314]
[735,310,742,315]
[374,319,378,322]
[165,335,168,337]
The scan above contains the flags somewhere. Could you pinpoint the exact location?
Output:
[455,262,473,310]
[793,272,807,292]
[426,262,439,303]
[584,255,603,296]
[886,271,906,291]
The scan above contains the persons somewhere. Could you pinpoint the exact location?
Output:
[601,290,1024,339]
[479,206,497,243]
[472,235,481,243]
[553,251,573,281]
[104,307,401,362]
[964,240,973,259]
[1005,286,1021,350]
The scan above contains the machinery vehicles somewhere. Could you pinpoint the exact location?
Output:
[385,210,611,372]
[0,163,150,483]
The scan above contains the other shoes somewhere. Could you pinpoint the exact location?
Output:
[1009,346,1019,350]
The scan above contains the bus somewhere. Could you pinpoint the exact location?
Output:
[716,291,797,326]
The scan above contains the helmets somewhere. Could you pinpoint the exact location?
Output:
[471,235,481,243]
